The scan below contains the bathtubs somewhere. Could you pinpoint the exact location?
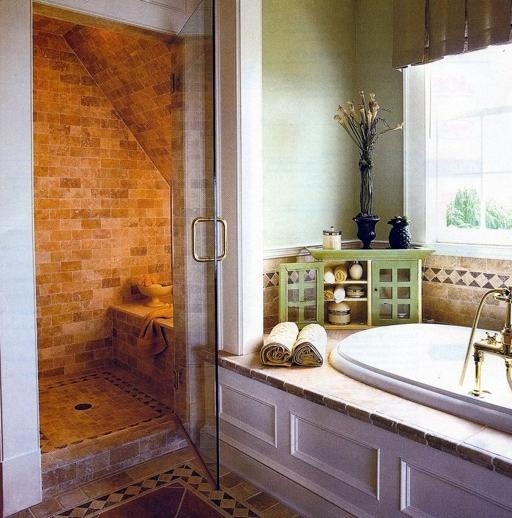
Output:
[329,321,512,435]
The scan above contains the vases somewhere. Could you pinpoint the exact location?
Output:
[387,218,413,248]
[353,216,379,249]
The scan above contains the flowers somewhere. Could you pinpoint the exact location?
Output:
[333,90,403,217]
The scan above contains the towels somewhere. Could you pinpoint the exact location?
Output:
[260,319,329,368]
[133,305,172,358]
[324,265,349,301]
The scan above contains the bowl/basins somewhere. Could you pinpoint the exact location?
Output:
[346,287,364,297]
[398,313,407,318]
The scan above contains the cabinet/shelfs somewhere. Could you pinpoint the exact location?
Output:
[280,252,425,330]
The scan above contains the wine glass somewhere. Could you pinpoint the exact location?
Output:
[136,284,172,308]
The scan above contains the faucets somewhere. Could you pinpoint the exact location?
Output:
[470,285,512,399]
